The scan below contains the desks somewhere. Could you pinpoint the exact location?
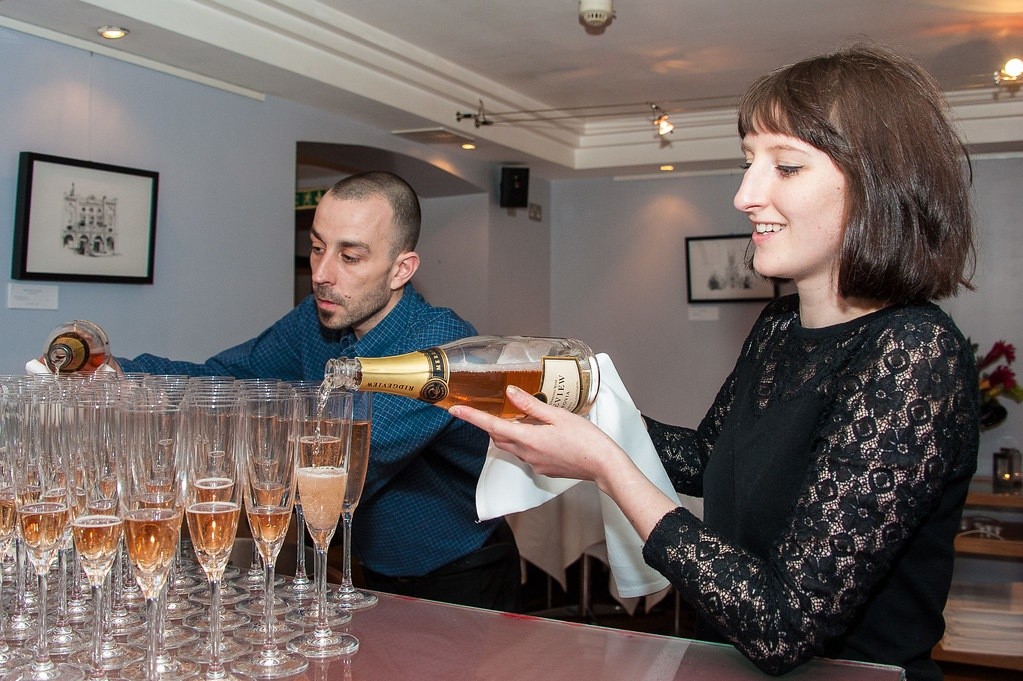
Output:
[0,566,905,681]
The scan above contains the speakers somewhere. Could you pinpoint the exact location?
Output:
[500,167,529,207]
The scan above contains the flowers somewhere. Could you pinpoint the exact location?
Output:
[966,333,1023,431]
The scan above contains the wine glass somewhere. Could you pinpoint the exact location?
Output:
[0,374,373,681]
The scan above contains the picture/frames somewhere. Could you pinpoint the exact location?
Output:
[11,151,160,285]
[684,232,780,305]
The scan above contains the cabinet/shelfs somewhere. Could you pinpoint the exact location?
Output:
[931,475,1022,671]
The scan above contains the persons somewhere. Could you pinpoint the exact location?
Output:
[448,45,982,681]
[39,170,500,596]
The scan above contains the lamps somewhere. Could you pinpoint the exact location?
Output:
[647,101,673,137]
[993,58,1023,85]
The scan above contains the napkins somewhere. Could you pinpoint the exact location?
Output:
[475,341,684,614]
[26,360,115,422]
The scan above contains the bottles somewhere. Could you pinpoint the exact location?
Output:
[323,335,601,424]
[43,320,111,375]
